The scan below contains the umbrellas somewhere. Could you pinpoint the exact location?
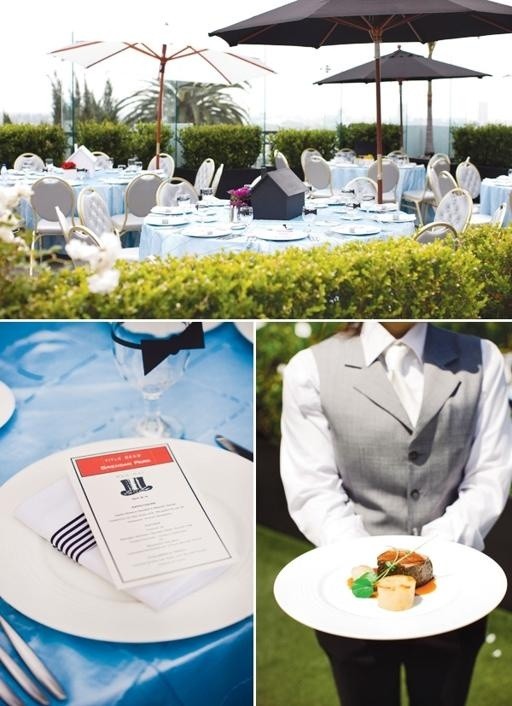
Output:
[42,20,280,172]
[313,45,494,160]
[204,0,512,205]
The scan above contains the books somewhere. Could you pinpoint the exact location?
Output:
[11,474,241,612]
[63,438,240,593]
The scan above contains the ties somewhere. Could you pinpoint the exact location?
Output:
[383,343,413,429]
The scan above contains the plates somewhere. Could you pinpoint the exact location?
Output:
[147,188,415,243]
[0,437,256,645]
[272,536,509,641]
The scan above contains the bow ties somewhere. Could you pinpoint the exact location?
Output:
[111,322,206,376]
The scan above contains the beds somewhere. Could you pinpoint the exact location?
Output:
[1,170,169,255]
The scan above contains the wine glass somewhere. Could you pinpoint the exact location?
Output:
[109,321,188,439]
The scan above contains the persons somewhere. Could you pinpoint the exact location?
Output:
[279,321,512,705]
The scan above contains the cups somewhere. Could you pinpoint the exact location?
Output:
[45,157,142,186]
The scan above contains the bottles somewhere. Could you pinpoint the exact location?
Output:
[1,163,9,186]
